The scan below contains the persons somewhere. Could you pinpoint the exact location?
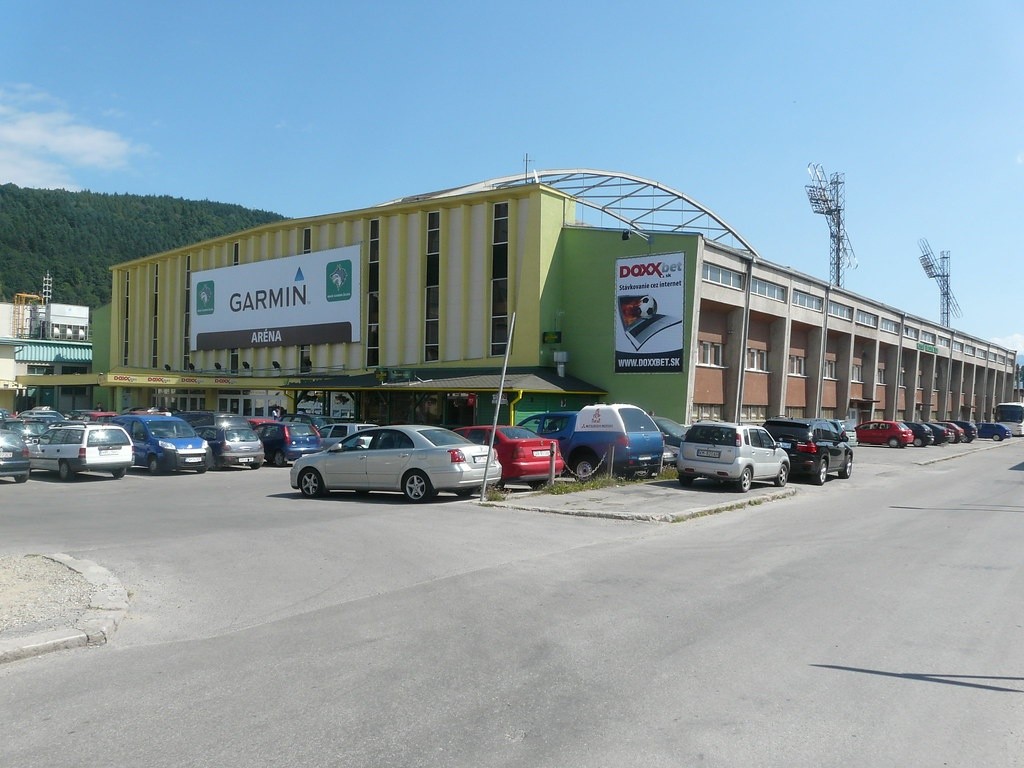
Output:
[93,401,105,412]
[648,410,656,417]
[272,407,283,421]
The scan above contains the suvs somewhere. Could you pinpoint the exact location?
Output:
[677,419,791,494]
[750,415,854,486]
[170,410,252,431]
[105,414,214,476]
[28,422,136,482]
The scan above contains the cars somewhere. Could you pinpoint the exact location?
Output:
[0,429,31,483]
[447,424,564,491]
[902,421,935,448]
[248,419,278,430]
[192,425,265,470]
[290,425,502,503]
[0,408,172,442]
[253,421,325,466]
[280,413,335,432]
[314,424,382,450]
[825,418,859,447]
[853,420,915,449]
[932,422,965,443]
[975,423,1012,441]
[921,422,955,446]
[662,446,679,466]
[650,415,689,448]
[940,420,978,443]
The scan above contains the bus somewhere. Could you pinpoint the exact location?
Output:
[993,401,1024,437]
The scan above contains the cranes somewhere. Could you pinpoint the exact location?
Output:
[917,236,964,328]
[804,160,860,290]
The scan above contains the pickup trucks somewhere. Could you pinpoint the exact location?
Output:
[512,403,665,482]
